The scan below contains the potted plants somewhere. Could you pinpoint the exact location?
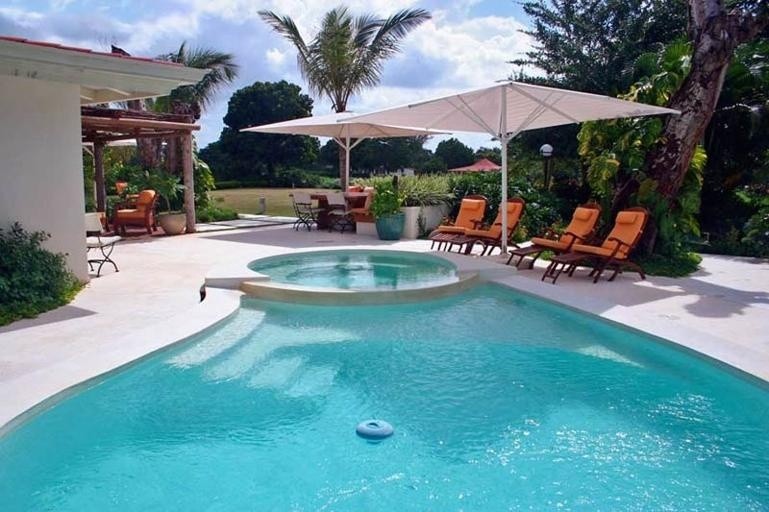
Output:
[138,166,187,234]
[368,181,408,240]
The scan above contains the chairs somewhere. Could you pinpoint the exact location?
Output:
[288,186,371,234]
[84,213,122,277]
[429,194,651,285]
[116,190,157,235]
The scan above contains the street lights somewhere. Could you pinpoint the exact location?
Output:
[540,144,554,190]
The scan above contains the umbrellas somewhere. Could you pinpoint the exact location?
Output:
[238,106,454,212]
[335,80,686,253]
